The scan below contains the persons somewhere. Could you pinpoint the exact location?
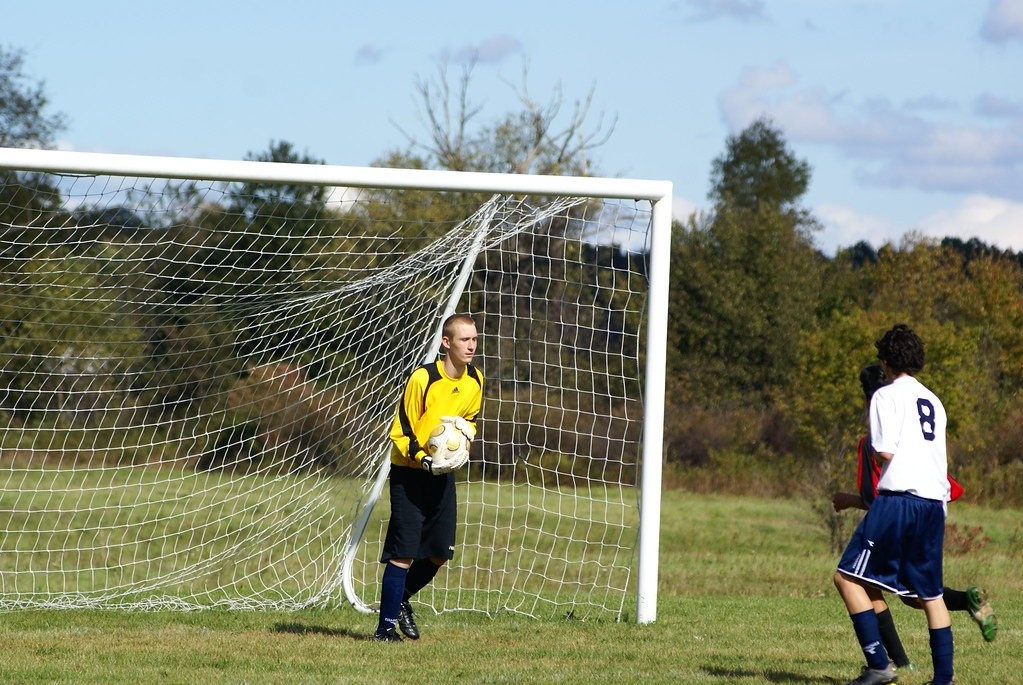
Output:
[373,314,484,643]
[833,322,998,683]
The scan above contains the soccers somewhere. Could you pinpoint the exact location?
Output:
[427,421,471,461]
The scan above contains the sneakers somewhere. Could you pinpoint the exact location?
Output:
[374,628,403,644]
[397,602,419,640]
[966,587,997,642]
[845,660,898,685]
[892,663,914,673]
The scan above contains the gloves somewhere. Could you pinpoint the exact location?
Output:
[420,445,469,476]
[440,416,474,442]
